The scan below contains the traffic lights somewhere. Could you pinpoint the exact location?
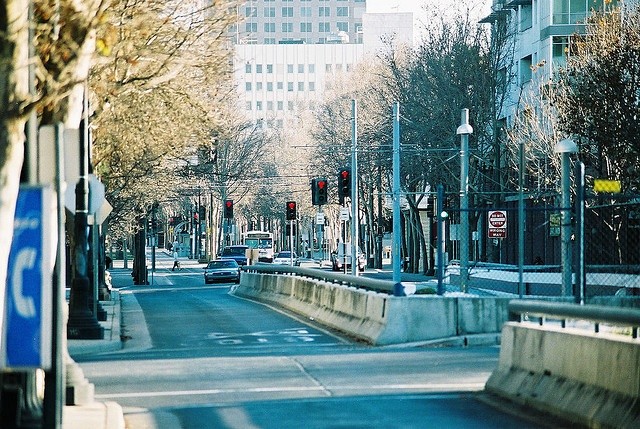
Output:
[286,201,296,220]
[224,199,233,219]
[198,205,205,220]
[314,178,328,205]
[338,166,352,197]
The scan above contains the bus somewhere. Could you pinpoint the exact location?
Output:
[245,231,275,264]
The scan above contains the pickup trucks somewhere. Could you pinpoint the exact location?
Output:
[330,243,366,272]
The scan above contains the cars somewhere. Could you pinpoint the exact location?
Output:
[203,260,242,284]
[274,251,300,268]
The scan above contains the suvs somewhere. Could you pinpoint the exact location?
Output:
[222,245,249,268]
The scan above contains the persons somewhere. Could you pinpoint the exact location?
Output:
[105,265,112,293]
[172,248,181,271]
